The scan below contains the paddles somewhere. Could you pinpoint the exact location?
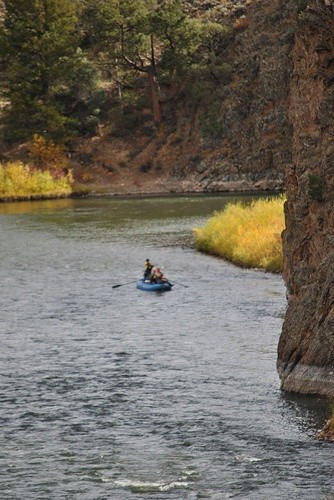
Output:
[112,278,150,288]
[162,277,189,287]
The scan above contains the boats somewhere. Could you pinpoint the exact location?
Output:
[136,277,171,291]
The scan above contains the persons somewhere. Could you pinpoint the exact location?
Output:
[140,259,165,286]
